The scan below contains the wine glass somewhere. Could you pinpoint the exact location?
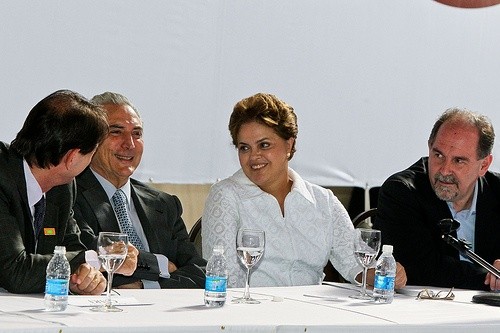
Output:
[89,232,129,312]
[231,228,265,304]
[349,228,381,300]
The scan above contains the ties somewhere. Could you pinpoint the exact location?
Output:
[35,195,45,242]
[112,191,145,251]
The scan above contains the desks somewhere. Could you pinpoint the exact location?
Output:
[0,284,500,333]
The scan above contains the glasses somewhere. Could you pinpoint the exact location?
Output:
[416,287,456,301]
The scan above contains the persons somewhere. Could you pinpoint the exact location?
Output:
[0,90,139,295]
[73,92,208,288]
[200,93,407,290]
[371,111,500,292]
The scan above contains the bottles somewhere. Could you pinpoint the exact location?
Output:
[372,245,396,304]
[43,246,70,312]
[204,245,228,308]
